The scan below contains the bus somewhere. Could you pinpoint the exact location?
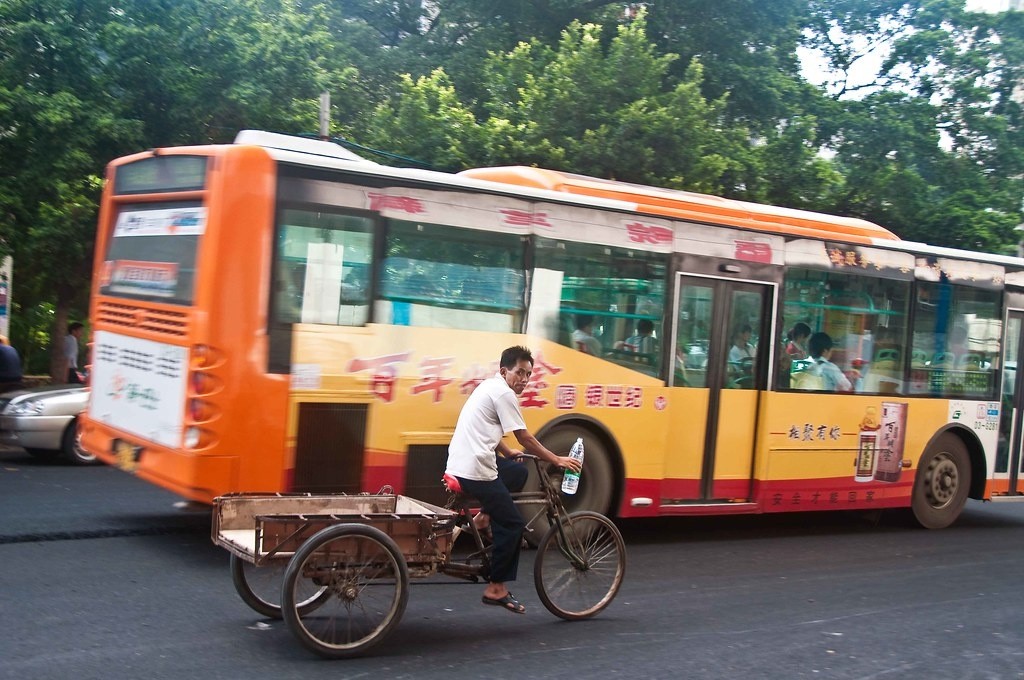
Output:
[80,128,1024,530]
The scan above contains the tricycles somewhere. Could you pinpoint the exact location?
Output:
[211,452,627,659]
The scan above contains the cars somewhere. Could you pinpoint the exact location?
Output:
[0,386,104,466]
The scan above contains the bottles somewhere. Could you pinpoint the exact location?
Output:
[853,405,876,482]
[561,437,585,494]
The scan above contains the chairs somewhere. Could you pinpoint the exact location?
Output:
[555,314,587,351]
[613,339,637,362]
[730,349,995,397]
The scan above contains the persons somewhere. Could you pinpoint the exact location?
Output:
[570,314,601,354]
[625,318,660,367]
[787,322,811,359]
[789,331,854,392]
[445,345,582,614]
[729,321,756,372]
[64,322,85,382]
[0,339,22,392]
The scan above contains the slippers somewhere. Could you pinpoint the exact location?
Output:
[461,524,493,541]
[482,591,527,614]
